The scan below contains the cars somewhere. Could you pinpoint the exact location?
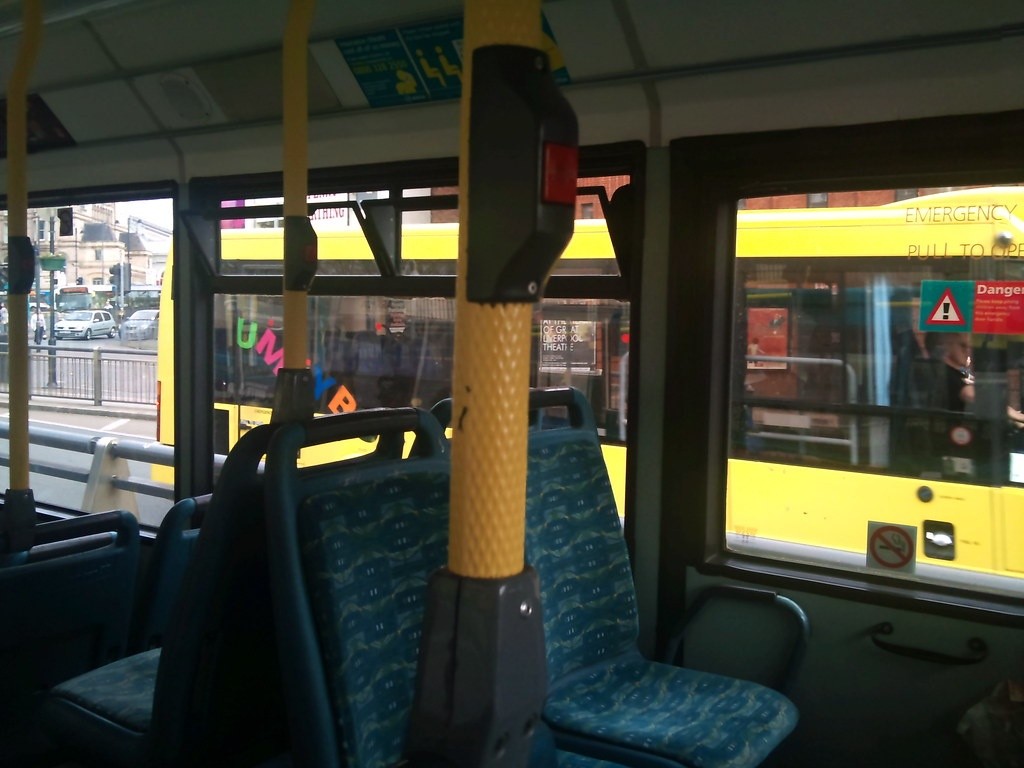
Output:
[119,309,159,340]
[52,309,117,340]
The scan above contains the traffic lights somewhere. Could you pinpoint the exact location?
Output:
[57,207,73,236]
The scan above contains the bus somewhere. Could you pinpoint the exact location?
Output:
[147,181,1024,581]
[54,285,92,311]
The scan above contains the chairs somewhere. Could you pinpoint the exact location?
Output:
[0,383,811,768]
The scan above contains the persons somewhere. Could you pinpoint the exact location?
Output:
[923,332,1024,423]
[30,309,46,342]
[0,308,8,332]
[90,299,114,309]
[747,338,765,367]
